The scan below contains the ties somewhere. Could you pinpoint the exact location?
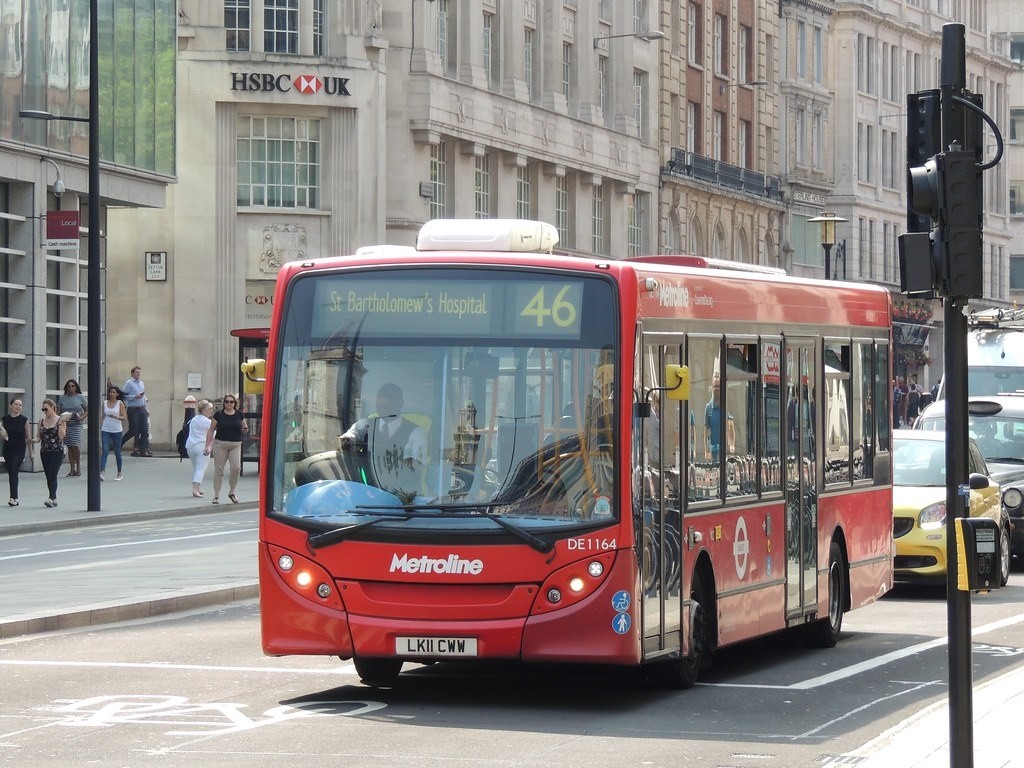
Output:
[381,424,389,441]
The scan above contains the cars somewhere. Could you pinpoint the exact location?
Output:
[909,392,1024,573]
[892,428,1013,595]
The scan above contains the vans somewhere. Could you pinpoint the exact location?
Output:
[917,303,1024,412]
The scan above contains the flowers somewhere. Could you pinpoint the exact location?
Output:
[892,303,934,322]
[892,351,934,367]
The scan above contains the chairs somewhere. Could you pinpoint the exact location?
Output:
[368,413,432,495]
[928,448,944,471]
[973,422,1024,457]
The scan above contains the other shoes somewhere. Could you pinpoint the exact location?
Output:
[229,494,238,503]
[100,474,104,481]
[141,451,150,457]
[115,476,123,480]
[44,500,57,508]
[67,473,81,478]
[192,489,204,498]
[212,497,220,504]
[8,500,19,506]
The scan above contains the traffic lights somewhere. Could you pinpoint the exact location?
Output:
[896,152,988,303]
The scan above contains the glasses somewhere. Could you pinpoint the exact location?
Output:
[68,385,75,388]
[41,408,48,412]
[225,401,234,404]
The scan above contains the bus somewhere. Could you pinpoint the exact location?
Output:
[240,217,895,691]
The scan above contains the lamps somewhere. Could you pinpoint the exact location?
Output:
[593,31,664,49]
[720,81,768,96]
[40,157,66,199]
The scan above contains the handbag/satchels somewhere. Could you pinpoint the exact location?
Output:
[139,417,152,440]
[176,421,189,457]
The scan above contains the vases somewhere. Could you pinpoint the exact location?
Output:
[892,316,928,325]
[893,362,926,367]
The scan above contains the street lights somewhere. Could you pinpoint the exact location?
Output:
[805,211,851,280]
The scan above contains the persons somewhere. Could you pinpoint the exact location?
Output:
[0,398,35,507]
[340,383,428,505]
[26,398,67,507]
[100,387,129,481]
[204,395,249,505]
[893,374,940,429]
[186,400,216,498]
[56,379,88,477]
[703,378,735,462]
[121,367,152,457]
